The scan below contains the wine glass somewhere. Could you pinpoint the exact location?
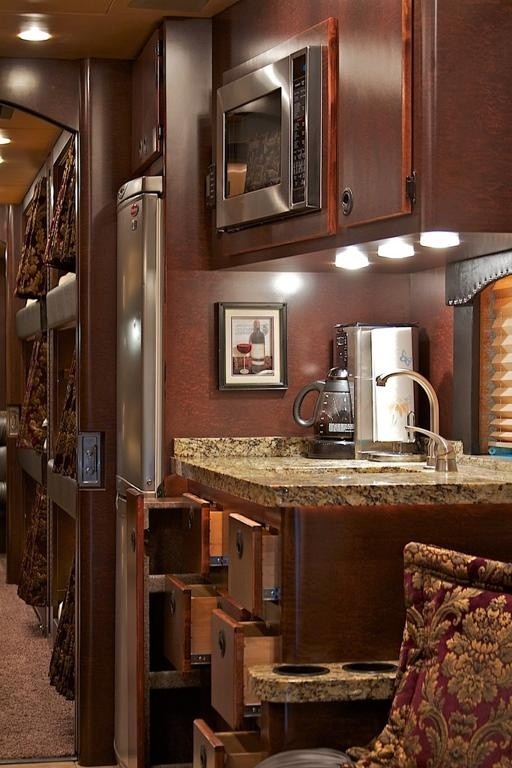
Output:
[238,337,253,374]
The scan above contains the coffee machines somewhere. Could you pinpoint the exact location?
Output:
[292,319,424,461]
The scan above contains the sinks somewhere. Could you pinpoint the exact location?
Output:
[266,465,418,478]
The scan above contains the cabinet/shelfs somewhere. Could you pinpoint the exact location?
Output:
[210,0,512,272]
[120,475,512,768]
[130,16,212,173]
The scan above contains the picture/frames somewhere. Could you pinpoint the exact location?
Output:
[215,301,289,391]
[77,430,105,491]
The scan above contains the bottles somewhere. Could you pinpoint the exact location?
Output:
[247,319,266,374]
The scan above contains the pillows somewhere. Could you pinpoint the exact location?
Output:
[340,542,512,768]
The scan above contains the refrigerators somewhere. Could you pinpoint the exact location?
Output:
[110,175,165,767]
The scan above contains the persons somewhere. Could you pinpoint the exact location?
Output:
[28,410,48,432]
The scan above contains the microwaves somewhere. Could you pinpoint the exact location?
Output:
[204,44,328,235]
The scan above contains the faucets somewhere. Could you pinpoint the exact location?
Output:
[375,368,441,468]
